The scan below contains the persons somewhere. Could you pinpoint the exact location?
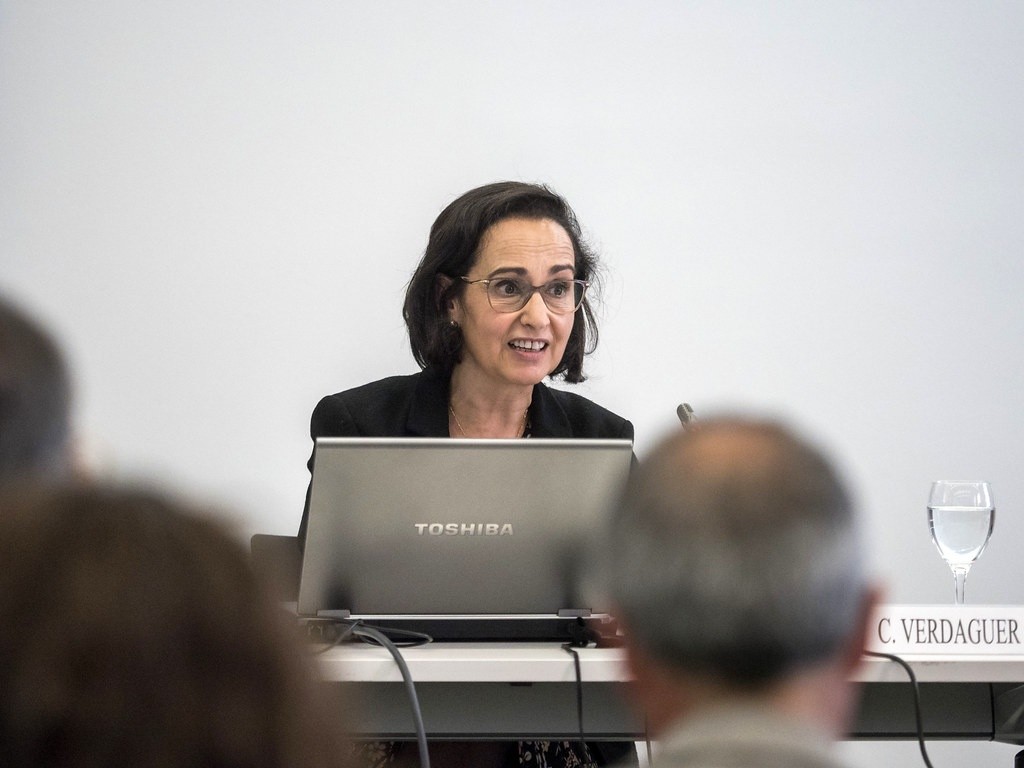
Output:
[297,181,644,767]
[600,414,884,767]
[0,297,347,768]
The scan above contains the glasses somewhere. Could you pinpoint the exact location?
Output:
[459,275,585,315]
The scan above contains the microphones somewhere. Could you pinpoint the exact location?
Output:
[676,402,703,434]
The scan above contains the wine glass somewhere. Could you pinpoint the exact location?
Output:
[926,479,996,605]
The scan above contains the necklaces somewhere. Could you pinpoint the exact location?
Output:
[450,405,528,439]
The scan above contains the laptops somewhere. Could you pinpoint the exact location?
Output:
[262,437,634,642]
